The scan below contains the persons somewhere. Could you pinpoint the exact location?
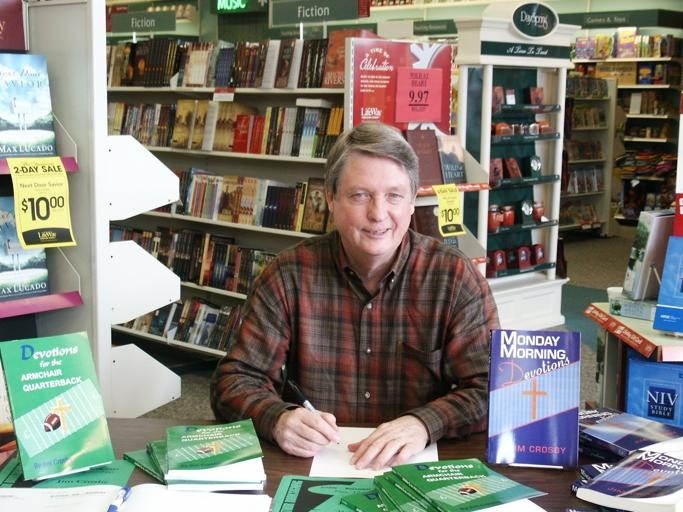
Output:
[207,122,502,470]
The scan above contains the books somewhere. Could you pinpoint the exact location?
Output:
[489,209,683,512]
[0,26,134,511]
[558,26,682,242]
[112,38,351,358]
[128,416,550,512]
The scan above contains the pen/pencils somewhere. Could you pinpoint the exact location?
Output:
[302,399,341,447]
[105,484,132,512]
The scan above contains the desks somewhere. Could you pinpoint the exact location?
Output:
[94,416,621,512]
